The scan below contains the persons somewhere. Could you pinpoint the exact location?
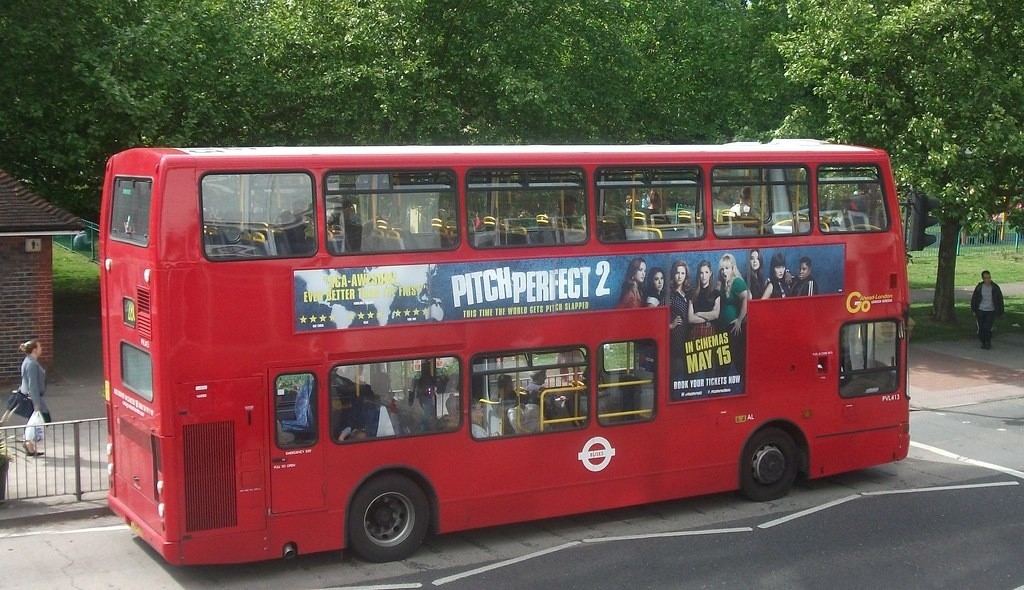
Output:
[17,339,51,456]
[635,191,673,227]
[728,185,766,219]
[616,246,818,400]
[274,200,378,256]
[845,184,877,225]
[547,194,584,232]
[971,270,1005,350]
[332,358,609,444]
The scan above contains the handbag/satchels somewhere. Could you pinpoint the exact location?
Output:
[6,391,34,417]
[24,410,46,442]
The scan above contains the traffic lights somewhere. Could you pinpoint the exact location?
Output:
[909,192,941,250]
[847,192,871,226]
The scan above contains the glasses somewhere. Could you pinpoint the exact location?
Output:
[985,276,990,278]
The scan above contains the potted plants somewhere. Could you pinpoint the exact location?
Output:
[0,431,33,506]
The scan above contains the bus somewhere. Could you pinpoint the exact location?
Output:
[98,137,912,565]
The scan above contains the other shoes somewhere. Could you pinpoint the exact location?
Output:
[981,340,991,349]
[23,443,44,456]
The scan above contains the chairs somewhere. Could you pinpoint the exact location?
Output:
[204,178,837,261]
